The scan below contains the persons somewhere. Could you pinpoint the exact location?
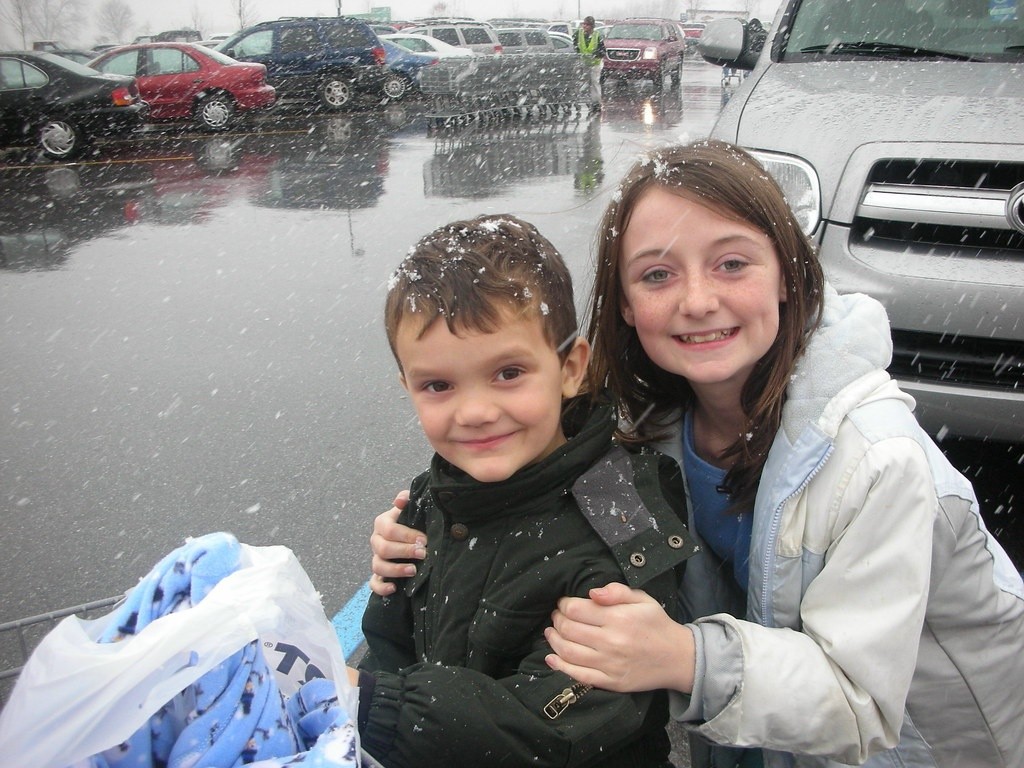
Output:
[573,16,605,111]
[744,18,768,77]
[344,214,701,768]
[372,141,1024,767]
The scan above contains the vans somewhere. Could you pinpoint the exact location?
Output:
[696,0,1023,450]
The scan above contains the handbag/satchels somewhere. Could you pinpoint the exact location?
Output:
[0,542,361,768]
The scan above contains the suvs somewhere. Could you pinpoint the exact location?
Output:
[599,16,685,88]
[198,16,390,112]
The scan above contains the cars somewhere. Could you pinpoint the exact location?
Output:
[0,50,151,162]
[376,38,447,102]
[29,6,707,80]
[82,42,275,134]
